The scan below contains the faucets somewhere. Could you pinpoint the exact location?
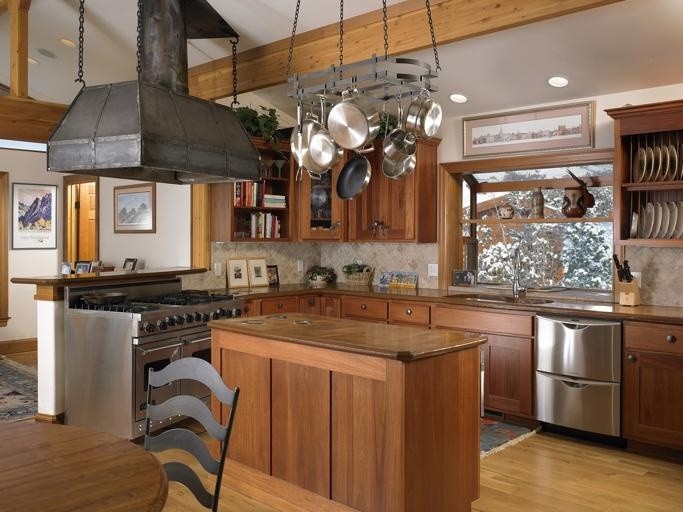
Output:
[510,247,528,299]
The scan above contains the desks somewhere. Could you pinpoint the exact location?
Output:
[2,421,168,512]
[206,311,488,511]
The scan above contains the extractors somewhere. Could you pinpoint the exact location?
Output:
[47,1,262,184]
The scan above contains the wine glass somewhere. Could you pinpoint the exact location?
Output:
[263,160,286,177]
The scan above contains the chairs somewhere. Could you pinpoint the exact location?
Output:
[143,356,240,511]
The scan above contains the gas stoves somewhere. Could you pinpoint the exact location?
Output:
[65,280,236,337]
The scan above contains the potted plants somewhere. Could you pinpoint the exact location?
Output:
[303,266,336,289]
[342,264,374,286]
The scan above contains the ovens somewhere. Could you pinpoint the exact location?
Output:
[66,324,213,439]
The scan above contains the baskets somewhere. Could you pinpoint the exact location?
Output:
[342,267,372,286]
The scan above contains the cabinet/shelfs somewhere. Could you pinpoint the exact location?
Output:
[620,135,683,246]
[347,142,414,241]
[623,320,682,460]
[434,325,532,419]
[321,297,340,317]
[302,152,340,236]
[233,147,294,240]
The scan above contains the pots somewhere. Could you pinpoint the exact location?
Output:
[290,87,442,199]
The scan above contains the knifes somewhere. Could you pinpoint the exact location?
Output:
[614,256,634,284]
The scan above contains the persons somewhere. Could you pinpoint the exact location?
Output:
[77,265,83,274]
[268,269,277,283]
[234,266,242,279]
[454,271,474,286]
[380,272,416,286]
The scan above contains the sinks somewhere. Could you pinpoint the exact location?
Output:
[443,293,554,305]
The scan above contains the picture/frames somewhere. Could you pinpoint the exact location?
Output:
[227,258,249,287]
[247,257,269,287]
[267,265,279,285]
[112,183,156,234]
[462,100,596,158]
[74,262,90,274]
[123,258,137,270]
[12,182,57,249]
[453,270,475,286]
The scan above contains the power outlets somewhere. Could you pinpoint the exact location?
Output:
[215,264,221,275]
[298,260,303,272]
[427,264,438,276]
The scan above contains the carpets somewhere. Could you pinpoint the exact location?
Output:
[479,409,539,458]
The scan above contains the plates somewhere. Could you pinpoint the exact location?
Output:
[633,144,678,184]
[630,201,683,240]
